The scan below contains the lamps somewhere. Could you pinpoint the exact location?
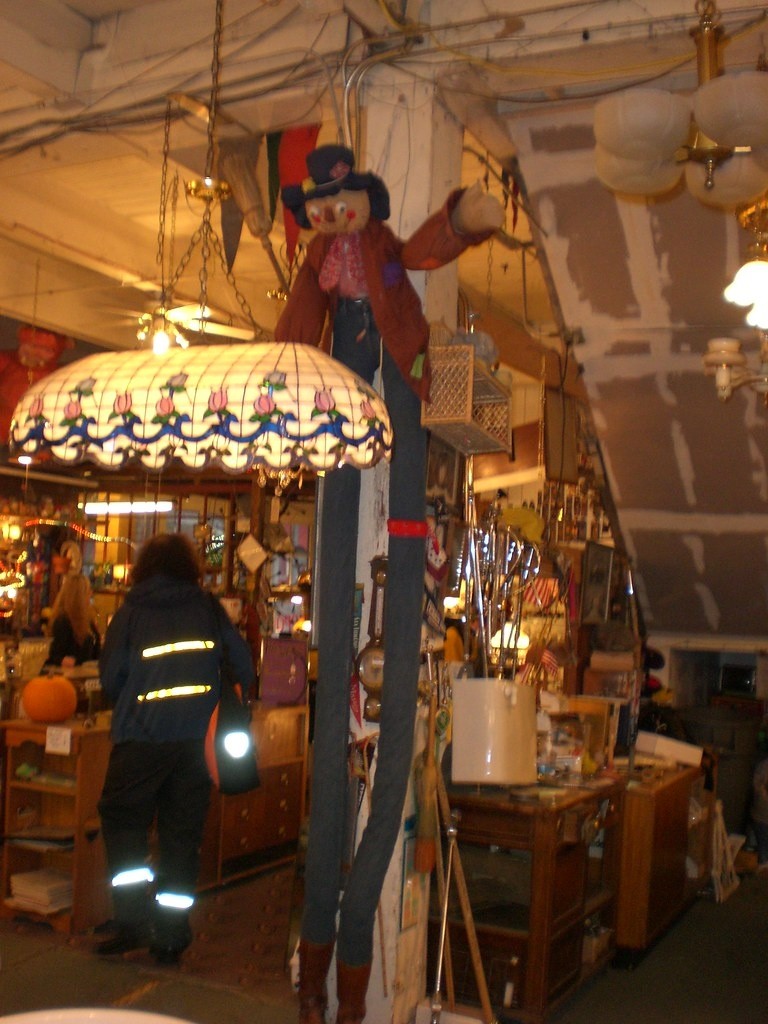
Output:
[189,319,263,343]
[149,315,171,353]
[704,330,768,406]
[593,0,768,207]
[722,191,768,330]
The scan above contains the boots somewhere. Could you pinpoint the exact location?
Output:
[80,868,155,956]
[149,887,196,964]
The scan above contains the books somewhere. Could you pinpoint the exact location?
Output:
[549,694,630,770]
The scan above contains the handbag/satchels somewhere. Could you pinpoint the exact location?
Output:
[207,590,262,796]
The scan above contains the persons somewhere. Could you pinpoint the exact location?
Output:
[276,144,505,403]
[97,534,235,962]
[41,574,101,678]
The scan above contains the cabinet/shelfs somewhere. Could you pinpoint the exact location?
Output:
[618,752,719,950]
[0,715,116,946]
[195,683,310,887]
[425,772,628,1024]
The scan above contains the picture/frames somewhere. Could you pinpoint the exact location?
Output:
[577,540,615,629]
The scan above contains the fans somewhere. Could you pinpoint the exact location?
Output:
[89,301,211,339]
